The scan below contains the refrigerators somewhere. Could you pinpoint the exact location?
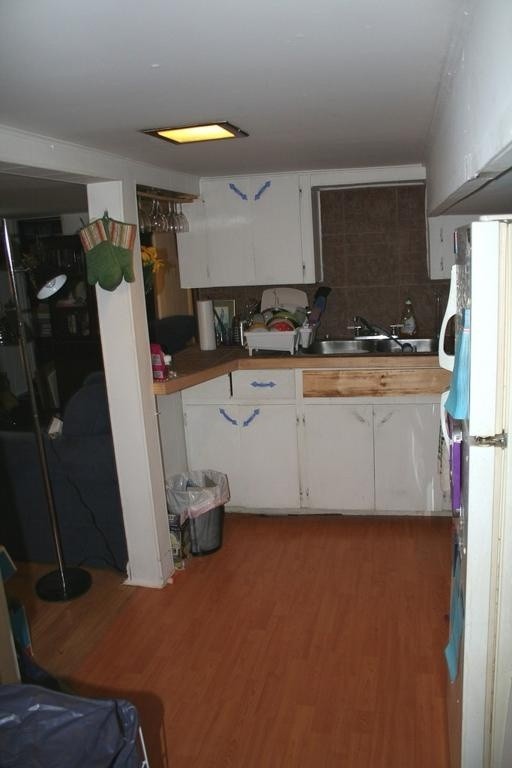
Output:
[444,213,512,768]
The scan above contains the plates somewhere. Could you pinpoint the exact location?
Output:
[252,307,299,331]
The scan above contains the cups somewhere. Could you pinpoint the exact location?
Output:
[222,328,233,346]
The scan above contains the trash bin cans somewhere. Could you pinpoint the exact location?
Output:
[166,470,230,555]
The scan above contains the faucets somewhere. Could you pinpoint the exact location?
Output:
[352,314,379,335]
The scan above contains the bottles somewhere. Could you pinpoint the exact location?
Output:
[398,298,418,339]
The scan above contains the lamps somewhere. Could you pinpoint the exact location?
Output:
[25,256,71,431]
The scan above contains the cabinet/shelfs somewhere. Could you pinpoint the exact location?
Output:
[302,401,441,514]
[182,369,301,510]
[427,215,474,281]
[205,173,306,288]
[24,235,106,404]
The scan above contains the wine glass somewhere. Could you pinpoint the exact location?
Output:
[138,190,189,234]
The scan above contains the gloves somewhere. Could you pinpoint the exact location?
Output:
[80,218,135,290]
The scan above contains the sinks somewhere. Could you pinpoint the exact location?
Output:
[298,336,377,355]
[375,337,440,356]
